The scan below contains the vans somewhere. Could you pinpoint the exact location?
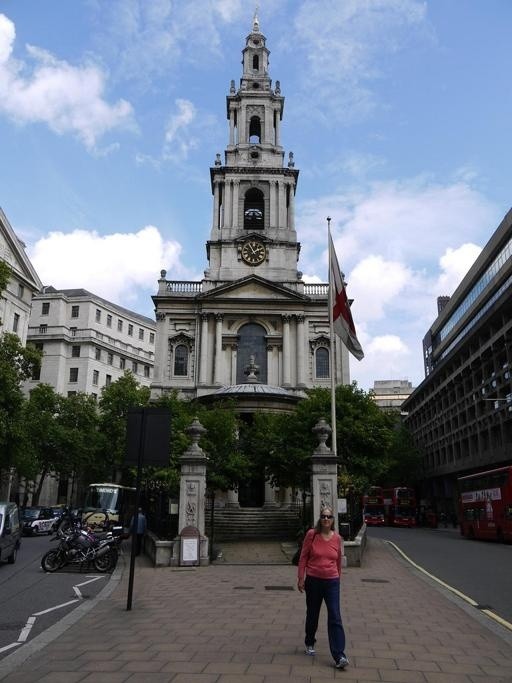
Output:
[0,501,25,568]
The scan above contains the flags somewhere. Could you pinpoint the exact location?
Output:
[327,227,364,362]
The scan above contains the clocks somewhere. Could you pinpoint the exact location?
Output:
[241,240,266,265]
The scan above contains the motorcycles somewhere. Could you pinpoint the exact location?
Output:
[39,504,132,575]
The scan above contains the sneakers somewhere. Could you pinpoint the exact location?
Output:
[336,656,349,668]
[305,644,316,654]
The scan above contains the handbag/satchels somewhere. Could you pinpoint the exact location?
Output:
[292,549,302,566]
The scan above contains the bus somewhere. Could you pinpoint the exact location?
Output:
[361,485,387,528]
[81,482,141,540]
[454,465,512,543]
[380,487,418,528]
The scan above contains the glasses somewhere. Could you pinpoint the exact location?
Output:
[320,514,333,519]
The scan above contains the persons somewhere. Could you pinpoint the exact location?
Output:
[296,504,350,668]
[128,507,148,556]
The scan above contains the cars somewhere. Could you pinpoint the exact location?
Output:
[20,503,66,537]
